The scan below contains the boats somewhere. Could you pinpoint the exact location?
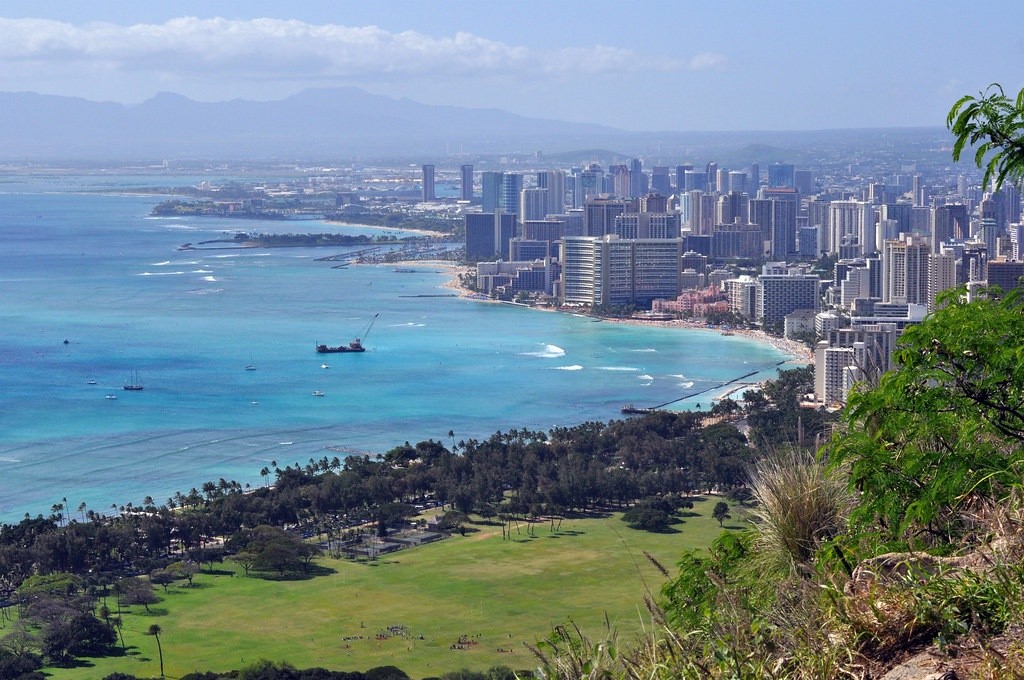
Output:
[315,340,366,353]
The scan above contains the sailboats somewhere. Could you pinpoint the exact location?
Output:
[123,367,145,390]
